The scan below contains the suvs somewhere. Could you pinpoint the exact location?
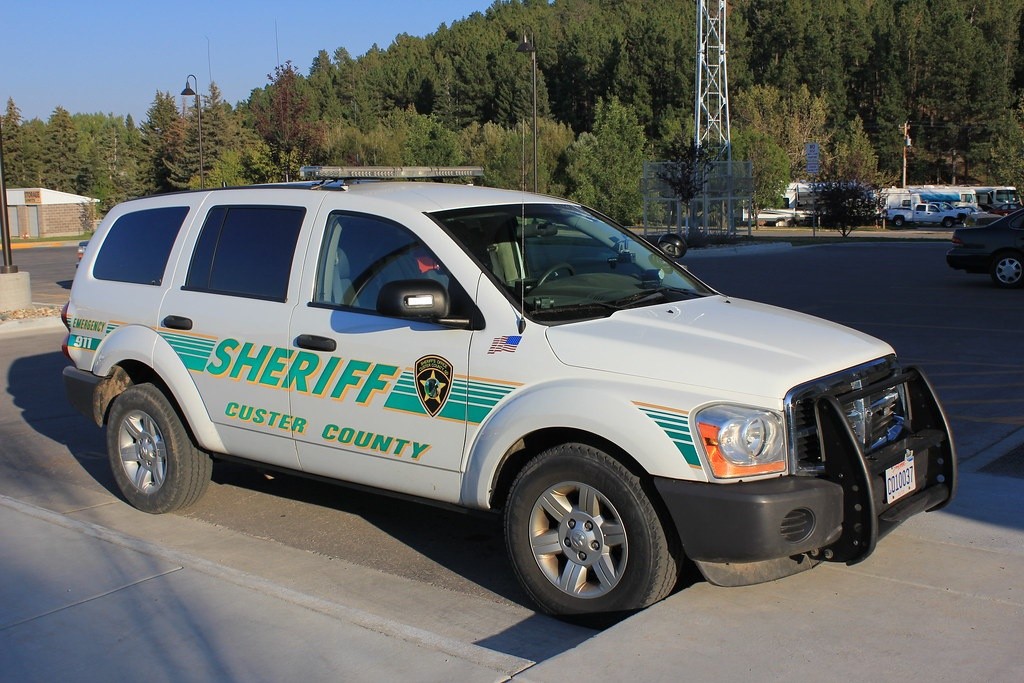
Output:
[59,164,957,627]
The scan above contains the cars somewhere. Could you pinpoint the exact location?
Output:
[943,211,1024,289]
[75,239,89,268]
[992,202,1023,216]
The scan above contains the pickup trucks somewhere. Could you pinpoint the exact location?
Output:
[929,200,969,224]
[886,203,957,228]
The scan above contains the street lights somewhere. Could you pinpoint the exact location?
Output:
[515,21,539,192]
[180,73,206,190]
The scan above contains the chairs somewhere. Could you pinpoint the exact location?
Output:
[392,240,449,288]
[337,245,403,308]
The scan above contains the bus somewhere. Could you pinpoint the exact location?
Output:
[972,186,1018,212]
